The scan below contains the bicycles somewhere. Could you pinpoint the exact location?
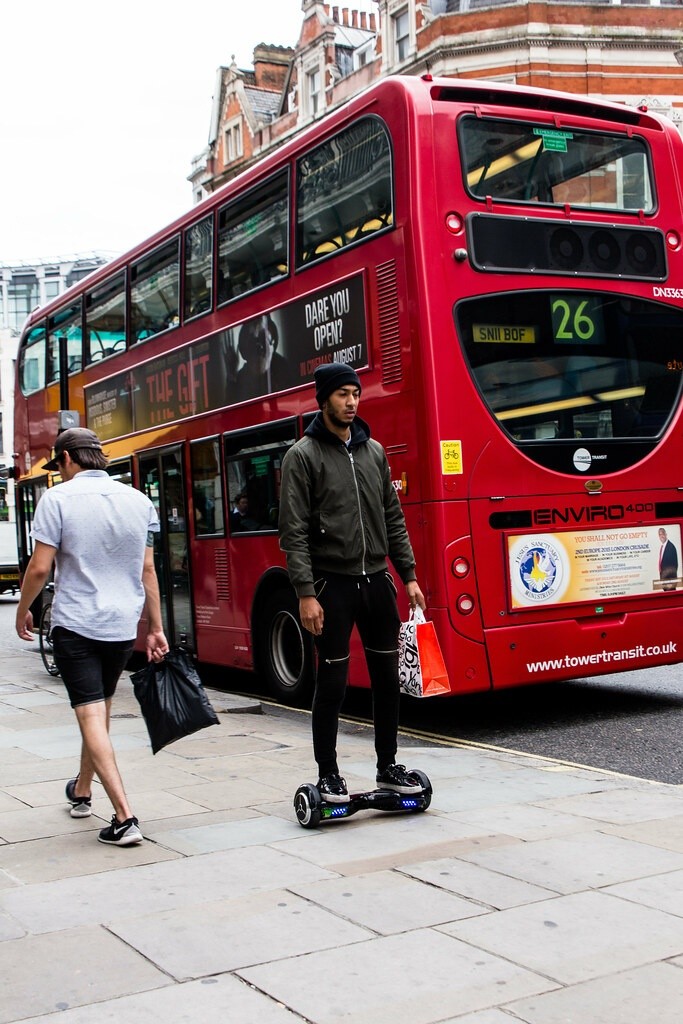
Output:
[39,583,60,678]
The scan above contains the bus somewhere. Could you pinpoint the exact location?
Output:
[10,70,683,701]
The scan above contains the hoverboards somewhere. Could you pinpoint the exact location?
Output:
[294,769,433,830]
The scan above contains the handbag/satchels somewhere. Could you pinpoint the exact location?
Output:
[129,645,221,756]
[398,602,452,697]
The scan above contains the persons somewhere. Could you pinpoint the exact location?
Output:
[12,427,173,840]
[230,493,253,531]
[279,364,428,805]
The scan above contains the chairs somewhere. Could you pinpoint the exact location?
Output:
[51,215,390,383]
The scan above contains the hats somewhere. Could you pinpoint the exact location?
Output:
[316,363,363,409]
[41,431,102,473]
[236,314,279,361]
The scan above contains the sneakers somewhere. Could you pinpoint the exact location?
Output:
[319,770,349,803]
[375,764,422,794]
[98,811,143,847]
[66,777,94,816]
